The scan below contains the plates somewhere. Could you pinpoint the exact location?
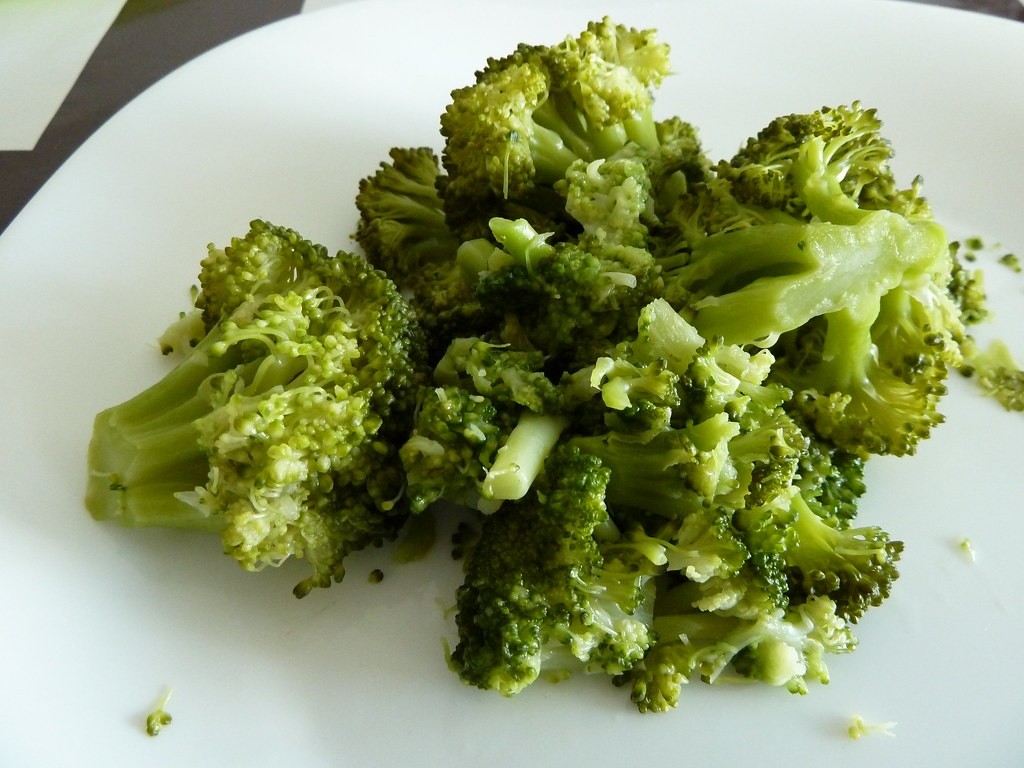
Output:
[0,0,1024,768]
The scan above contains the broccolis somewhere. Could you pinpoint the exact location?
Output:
[84,15,1024,735]
